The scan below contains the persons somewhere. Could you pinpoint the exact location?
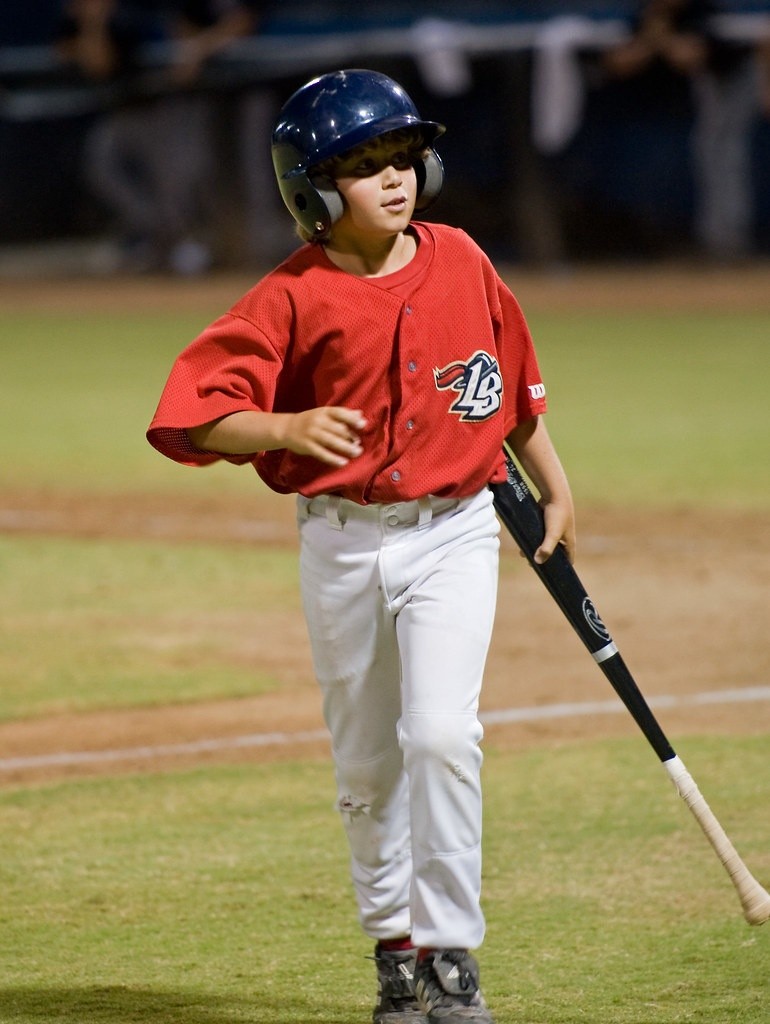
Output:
[77,0,250,278]
[147,69,575,1024]
[577,0,713,258]
[749,26,770,248]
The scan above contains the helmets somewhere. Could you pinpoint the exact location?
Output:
[273,69,444,238]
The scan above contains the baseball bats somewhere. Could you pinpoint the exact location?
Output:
[485,443,770,929]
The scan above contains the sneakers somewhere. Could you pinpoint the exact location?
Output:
[372,944,430,1024]
[413,948,492,1024]
